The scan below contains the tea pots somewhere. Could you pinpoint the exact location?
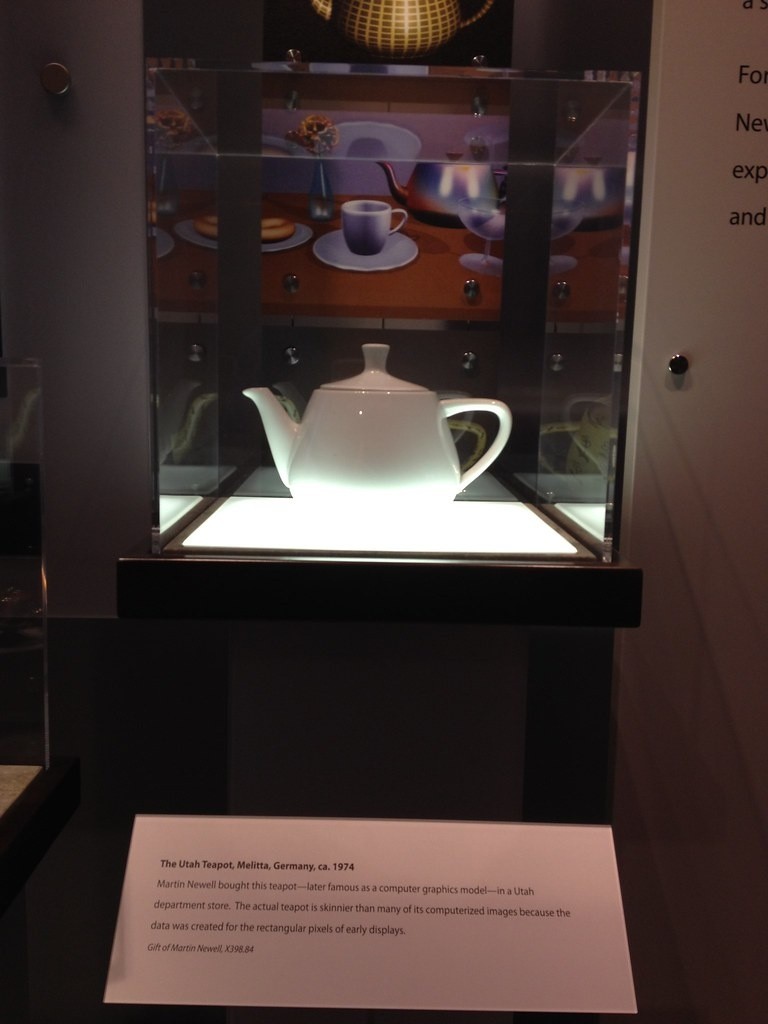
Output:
[243,343,512,501]
[377,152,508,228]
[551,155,626,230]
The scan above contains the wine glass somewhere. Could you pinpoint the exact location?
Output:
[456,198,506,277]
[549,199,586,275]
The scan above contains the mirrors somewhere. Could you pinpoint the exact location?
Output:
[141,0,653,566]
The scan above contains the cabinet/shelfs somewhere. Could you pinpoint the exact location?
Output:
[118,533,643,835]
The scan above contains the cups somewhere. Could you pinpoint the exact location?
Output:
[342,200,407,256]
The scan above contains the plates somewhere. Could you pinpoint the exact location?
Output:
[312,229,419,272]
[172,219,313,253]
